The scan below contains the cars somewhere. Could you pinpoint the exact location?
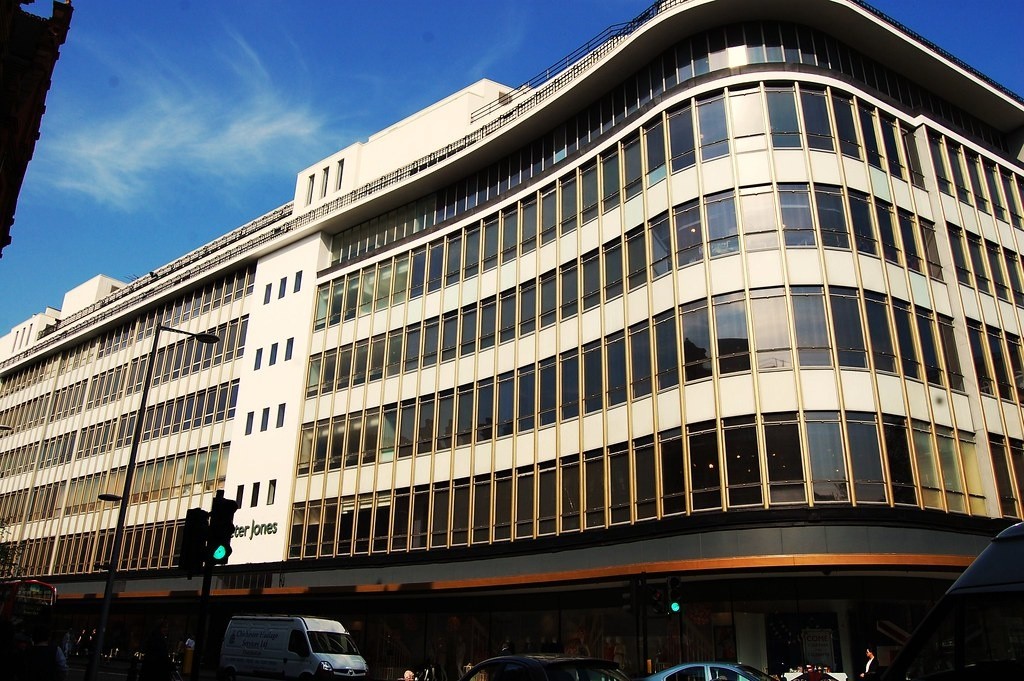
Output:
[631,662,779,681]
[458,653,632,681]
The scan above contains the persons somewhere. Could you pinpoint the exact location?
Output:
[14,625,69,674]
[438,635,466,681]
[404,671,414,681]
[176,635,195,654]
[139,620,178,681]
[860,647,879,681]
[503,636,565,654]
[578,636,627,669]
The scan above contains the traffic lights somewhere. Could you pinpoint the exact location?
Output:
[666,576,684,616]
[208,496,237,565]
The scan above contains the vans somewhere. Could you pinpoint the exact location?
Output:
[219,616,369,681]
[867,521,1023,681]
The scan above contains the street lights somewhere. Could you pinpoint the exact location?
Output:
[88,321,220,681]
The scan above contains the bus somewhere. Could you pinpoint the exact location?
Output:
[0,579,59,653]
